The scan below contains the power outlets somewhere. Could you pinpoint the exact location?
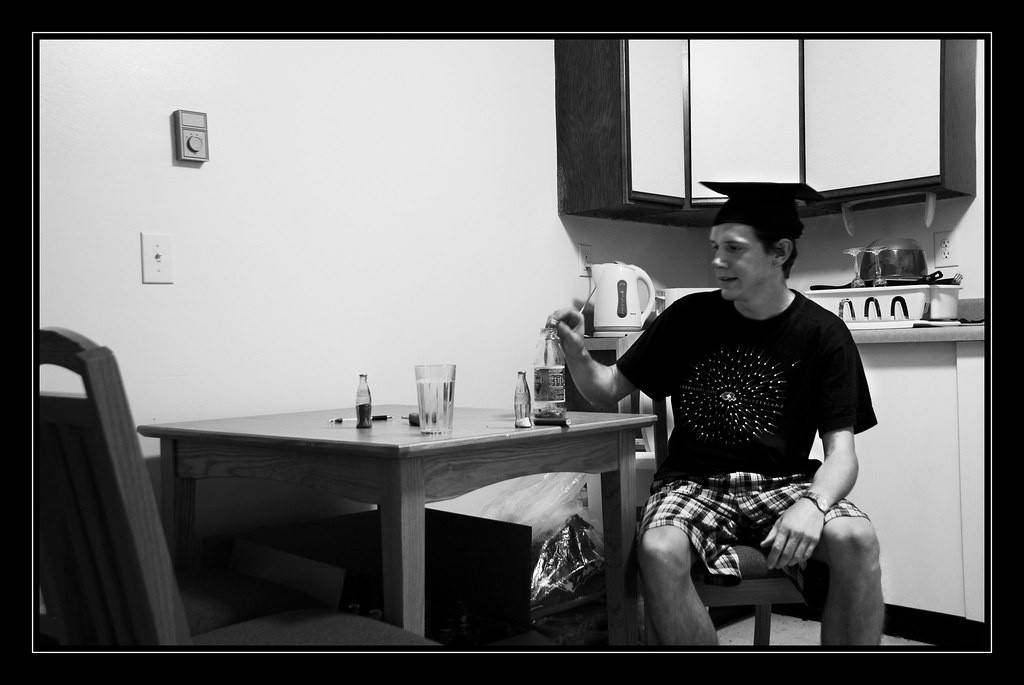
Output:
[937,229,959,268]
[140,231,173,284]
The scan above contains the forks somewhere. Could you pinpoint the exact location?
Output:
[953,274,964,284]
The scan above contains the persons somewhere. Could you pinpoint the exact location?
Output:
[546,185,886,646]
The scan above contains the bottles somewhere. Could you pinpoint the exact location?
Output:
[513,371,532,428]
[534,328,565,425]
[356,374,372,429]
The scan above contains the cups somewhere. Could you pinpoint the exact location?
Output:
[414,365,456,435]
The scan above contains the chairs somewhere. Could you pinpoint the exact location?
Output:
[657,396,807,645]
[39,326,435,648]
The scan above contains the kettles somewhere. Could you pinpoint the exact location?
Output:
[588,260,657,335]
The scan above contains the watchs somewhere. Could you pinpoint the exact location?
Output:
[799,491,832,515]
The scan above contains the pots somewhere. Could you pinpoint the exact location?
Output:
[812,238,942,290]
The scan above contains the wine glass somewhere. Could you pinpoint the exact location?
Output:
[865,247,889,287]
[843,246,868,288]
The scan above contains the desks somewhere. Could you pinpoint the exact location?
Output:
[137,404,656,638]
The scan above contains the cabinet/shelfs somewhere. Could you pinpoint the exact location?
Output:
[554,39,978,228]
